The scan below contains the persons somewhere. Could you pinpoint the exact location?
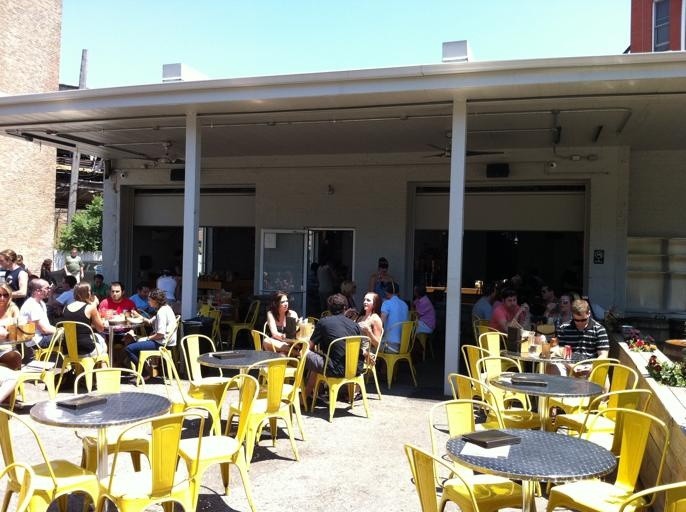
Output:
[0,247,182,409]
[471,281,610,422]
[260,257,437,410]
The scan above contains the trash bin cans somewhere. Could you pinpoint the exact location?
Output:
[182,317,215,367]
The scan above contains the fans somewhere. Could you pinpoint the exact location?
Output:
[423,130,505,158]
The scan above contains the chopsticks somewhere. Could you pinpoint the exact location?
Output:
[108,319,126,324]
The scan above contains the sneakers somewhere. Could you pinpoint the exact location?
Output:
[307,396,329,408]
[131,374,152,384]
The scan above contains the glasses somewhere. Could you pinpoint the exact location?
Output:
[0,293,9,298]
[558,301,568,305]
[572,318,587,323]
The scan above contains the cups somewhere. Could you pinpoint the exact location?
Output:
[301,323,315,338]
[207,298,212,305]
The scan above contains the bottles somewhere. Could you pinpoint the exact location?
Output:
[564,344,572,360]
[541,341,550,359]
[550,337,558,347]
[529,331,535,353]
[0,320,35,341]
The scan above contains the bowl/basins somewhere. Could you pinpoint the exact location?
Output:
[127,318,142,323]
[113,314,125,320]
